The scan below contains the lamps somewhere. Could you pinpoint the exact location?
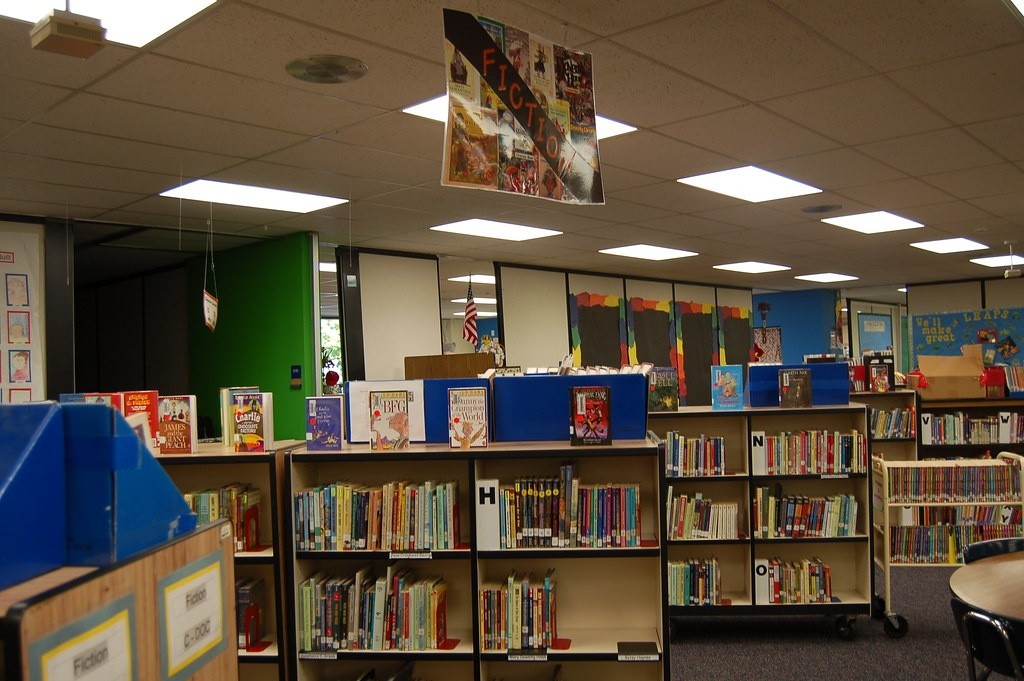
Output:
[30,0,107,61]
[1004,239,1021,279]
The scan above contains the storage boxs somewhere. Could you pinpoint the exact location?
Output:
[907,344,1006,402]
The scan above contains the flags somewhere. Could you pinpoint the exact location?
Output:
[462,272,479,348]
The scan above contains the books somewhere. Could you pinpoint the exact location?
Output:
[182,481,264,554]
[369,390,411,451]
[711,364,744,412]
[873,451,1022,504]
[648,366,680,413]
[476,464,641,551]
[569,386,613,447]
[298,559,447,654]
[666,485,740,540]
[753,482,858,539]
[294,478,460,551]
[751,429,868,476]
[60,390,162,459]
[235,574,268,648]
[779,368,813,409]
[985,365,1024,400]
[220,386,275,453]
[158,395,197,455]
[305,395,344,452]
[480,354,655,380]
[667,554,722,606]
[870,402,916,439]
[663,430,726,477]
[921,411,1024,446]
[447,386,490,448]
[552,663,563,681]
[479,567,557,651]
[356,660,415,681]
[755,555,833,604]
[845,349,908,393]
[747,361,783,367]
[802,353,837,364]
[876,505,1024,563]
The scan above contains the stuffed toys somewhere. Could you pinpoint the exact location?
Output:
[479,335,504,368]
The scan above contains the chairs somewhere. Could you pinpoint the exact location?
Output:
[950,598,1024,681]
[962,537,1024,566]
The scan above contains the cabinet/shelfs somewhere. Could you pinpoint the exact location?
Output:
[152,386,1024,681]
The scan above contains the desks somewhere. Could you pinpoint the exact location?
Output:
[948,551,1024,620]
